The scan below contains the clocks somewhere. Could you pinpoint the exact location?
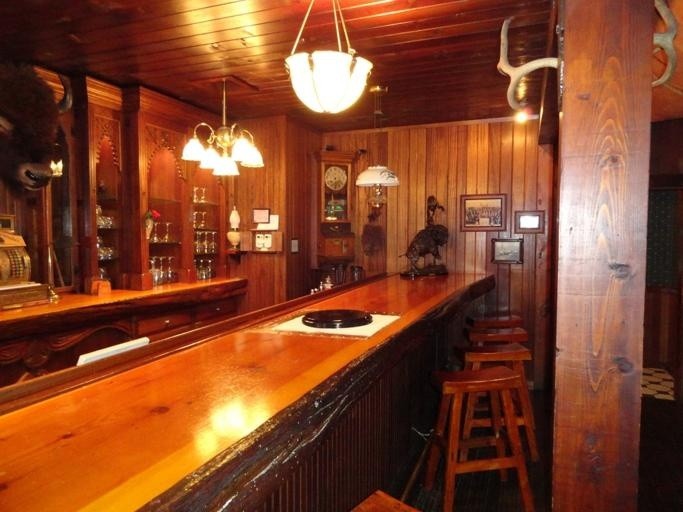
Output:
[320,161,352,224]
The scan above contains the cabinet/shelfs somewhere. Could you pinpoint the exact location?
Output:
[134,294,240,343]
[79,77,229,289]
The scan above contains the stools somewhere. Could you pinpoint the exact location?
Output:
[425,315,541,511]
[349,489,420,512]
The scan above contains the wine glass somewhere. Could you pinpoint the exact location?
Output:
[149,256,176,287]
[193,212,208,229]
[195,231,218,254]
[193,188,207,203]
[151,222,173,243]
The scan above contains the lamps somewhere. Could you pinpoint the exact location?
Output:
[284,0,373,114]
[356,165,399,214]
[226,205,241,251]
[180,78,264,177]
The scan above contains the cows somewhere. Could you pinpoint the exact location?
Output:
[0,62,72,193]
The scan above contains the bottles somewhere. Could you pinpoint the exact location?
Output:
[194,260,213,279]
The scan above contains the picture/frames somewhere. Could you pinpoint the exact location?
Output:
[515,211,545,233]
[460,195,506,230]
[251,208,271,224]
[491,238,524,264]
